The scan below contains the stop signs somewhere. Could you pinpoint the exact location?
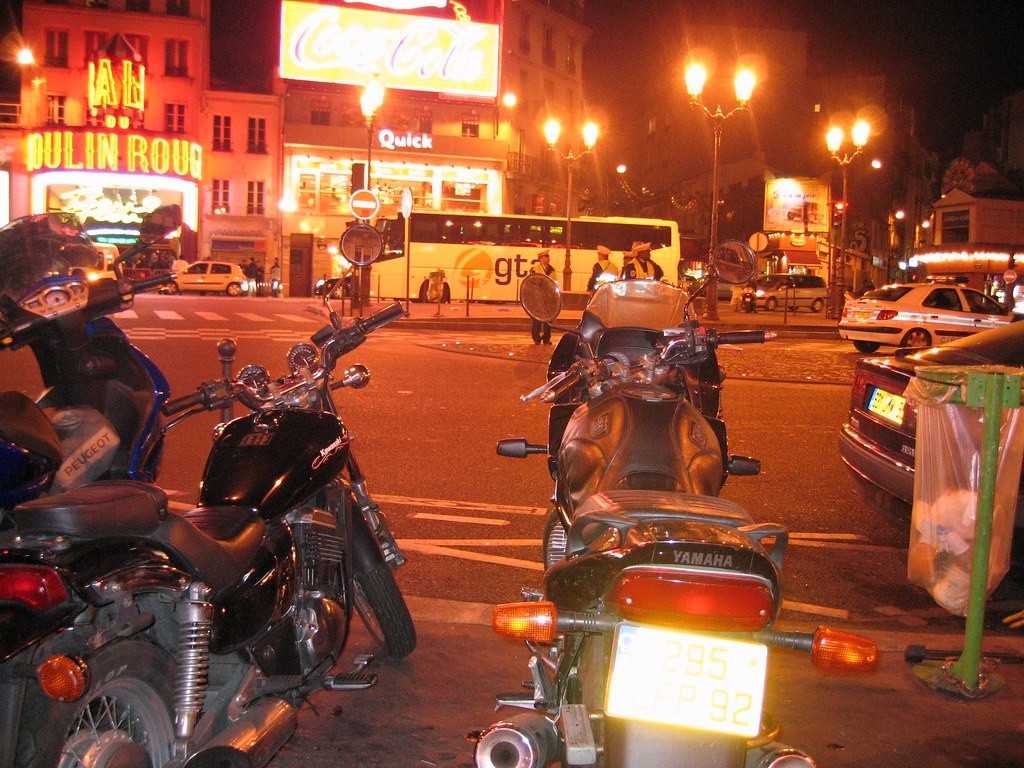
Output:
[349,189,380,220]
[1003,270,1017,283]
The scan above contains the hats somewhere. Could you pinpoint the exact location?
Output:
[597,245,612,255]
[537,249,550,256]
[623,240,652,257]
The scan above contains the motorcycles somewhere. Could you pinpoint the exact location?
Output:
[0,304,417,767]
[0,204,184,511]
[466,238,877,768]
[740,286,755,314]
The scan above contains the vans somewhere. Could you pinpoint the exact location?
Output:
[89,240,125,283]
[756,273,828,312]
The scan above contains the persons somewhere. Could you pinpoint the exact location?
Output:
[620,242,664,282]
[586,245,619,295]
[970,294,987,312]
[172,255,189,295]
[530,249,557,345]
[731,283,742,304]
[743,277,758,312]
[238,257,265,297]
[198,257,213,295]
[270,257,281,297]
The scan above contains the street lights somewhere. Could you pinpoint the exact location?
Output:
[360,81,385,310]
[542,118,599,291]
[825,121,869,319]
[685,63,755,321]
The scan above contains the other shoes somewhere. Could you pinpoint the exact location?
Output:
[535,340,541,345]
[543,340,552,345]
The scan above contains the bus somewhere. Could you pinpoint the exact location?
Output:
[369,209,681,303]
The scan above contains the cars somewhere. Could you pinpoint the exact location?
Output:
[156,260,249,296]
[838,282,1024,353]
[313,274,360,299]
[836,320,1024,574]
